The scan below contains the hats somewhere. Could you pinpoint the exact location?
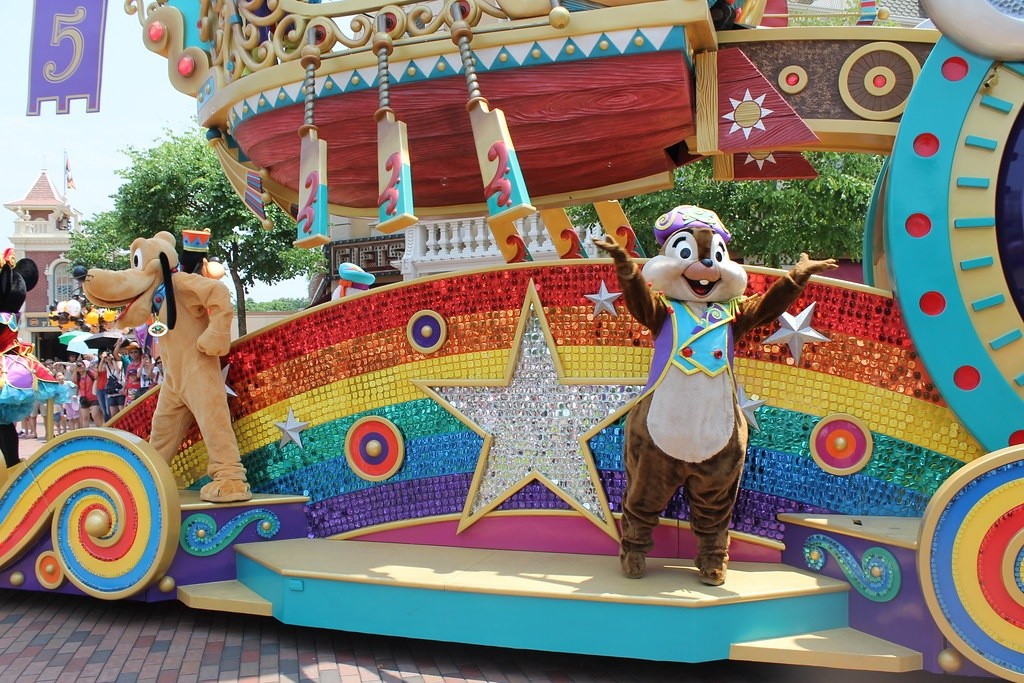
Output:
[128,369,138,375]
[126,341,140,349]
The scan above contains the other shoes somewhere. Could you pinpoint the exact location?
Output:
[18,431,37,439]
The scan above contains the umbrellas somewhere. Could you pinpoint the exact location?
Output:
[67,334,98,354]
[58,332,81,345]
[84,332,130,348]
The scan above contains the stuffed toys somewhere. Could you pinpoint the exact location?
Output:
[331,263,375,301]
[591,205,840,583]
[74,232,254,502]
[0,249,68,466]
[180,229,225,281]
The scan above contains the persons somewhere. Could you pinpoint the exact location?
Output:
[19,338,162,439]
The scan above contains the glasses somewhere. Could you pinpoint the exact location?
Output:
[130,350,138,354]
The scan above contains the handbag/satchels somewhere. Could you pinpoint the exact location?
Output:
[105,374,123,395]
